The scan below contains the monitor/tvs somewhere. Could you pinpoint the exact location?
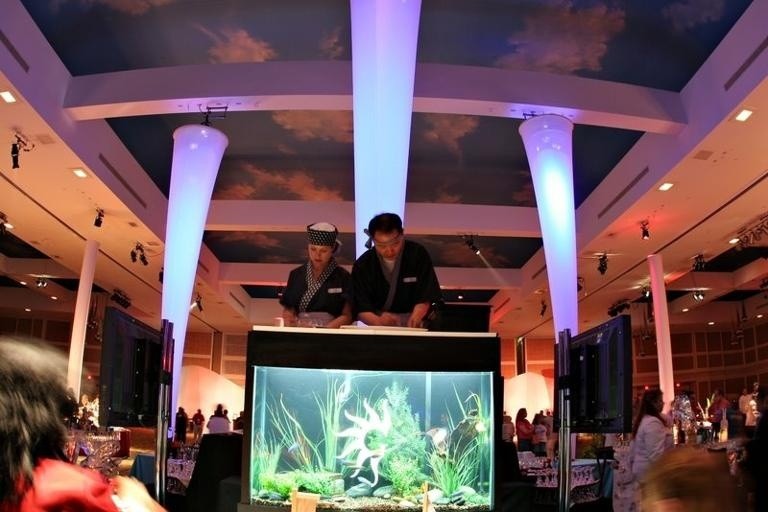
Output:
[553,314,632,433]
[98,305,175,428]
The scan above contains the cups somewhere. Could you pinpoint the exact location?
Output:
[516,451,539,468]
[291,488,319,512]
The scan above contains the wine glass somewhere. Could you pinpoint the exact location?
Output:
[537,466,595,488]
[167,458,195,480]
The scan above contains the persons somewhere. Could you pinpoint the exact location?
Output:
[206,403,245,433]
[278,221,355,329]
[1,336,120,512]
[632,387,675,480]
[741,383,768,511]
[192,408,205,443]
[62,387,100,427]
[666,381,760,444]
[503,408,553,456]
[350,211,443,329]
[174,404,188,444]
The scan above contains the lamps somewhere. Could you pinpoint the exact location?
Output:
[347,1,427,321]
[159,123,230,437]
[94,206,105,227]
[129,244,154,271]
[531,220,710,320]
[8,130,39,173]
[734,216,768,251]
[516,115,584,461]
[194,288,206,311]
